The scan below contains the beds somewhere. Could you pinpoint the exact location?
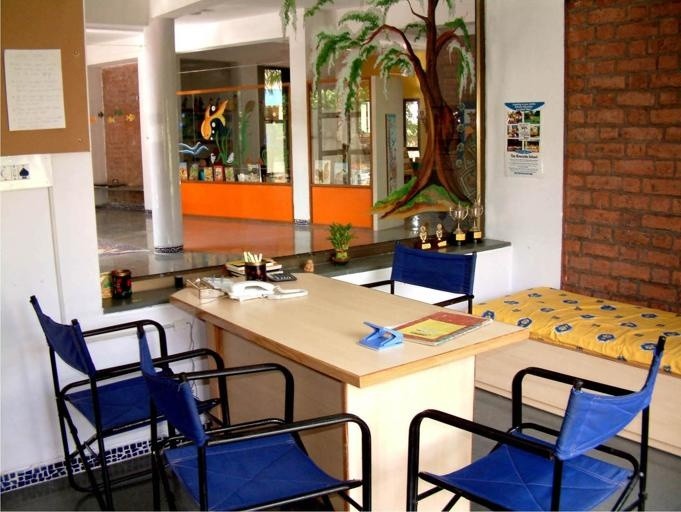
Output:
[463,285,681,459]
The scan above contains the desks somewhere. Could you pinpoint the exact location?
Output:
[169,273,530,512]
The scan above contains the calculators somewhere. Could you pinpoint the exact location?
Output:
[265,269,297,282]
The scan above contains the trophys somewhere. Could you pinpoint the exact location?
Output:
[449,200,470,245]
[468,199,485,244]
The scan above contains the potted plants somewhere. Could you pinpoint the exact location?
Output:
[327,222,353,265]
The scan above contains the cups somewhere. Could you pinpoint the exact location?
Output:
[110,269,133,301]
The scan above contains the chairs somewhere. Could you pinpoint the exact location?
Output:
[406,336,666,512]
[135,322,372,512]
[29,294,231,512]
[356,239,477,315]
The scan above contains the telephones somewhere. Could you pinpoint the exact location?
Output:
[225,280,275,302]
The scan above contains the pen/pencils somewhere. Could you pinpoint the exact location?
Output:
[243,251,263,263]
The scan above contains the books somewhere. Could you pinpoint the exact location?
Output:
[334,163,349,185]
[315,160,330,184]
[226,258,284,278]
[384,311,494,348]
[179,162,235,183]
[241,162,262,182]
[271,160,289,183]
[350,163,371,186]
